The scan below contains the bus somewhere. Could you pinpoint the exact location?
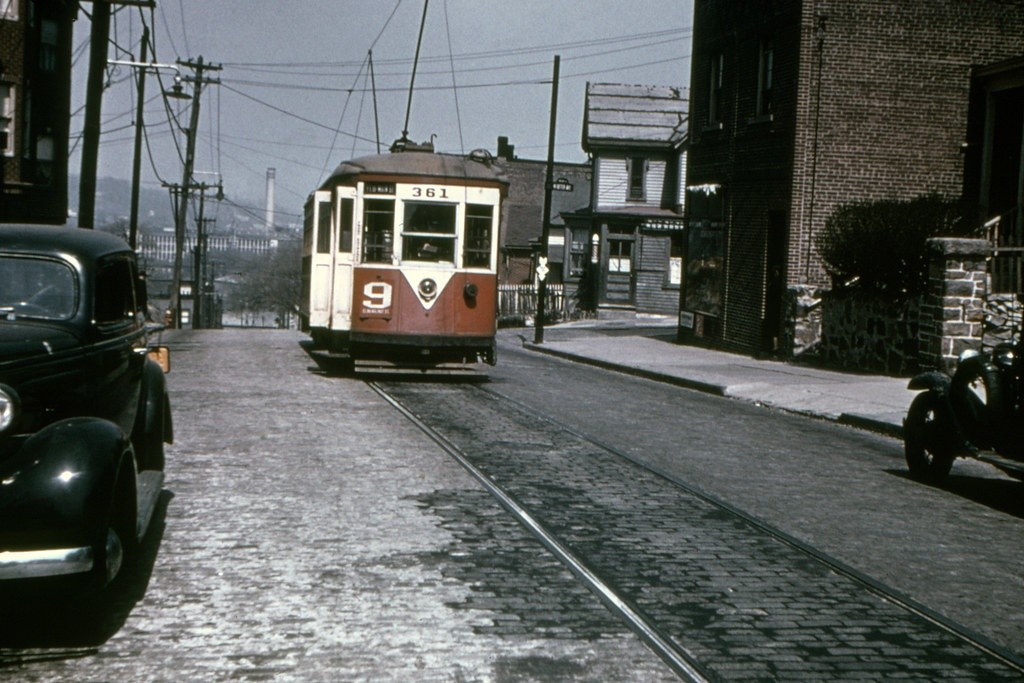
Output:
[299,133,512,384]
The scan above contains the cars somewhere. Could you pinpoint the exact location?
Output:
[1,222,178,647]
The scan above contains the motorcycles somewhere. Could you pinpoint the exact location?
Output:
[905,318,1024,492]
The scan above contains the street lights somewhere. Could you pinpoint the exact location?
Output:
[104,55,184,249]
